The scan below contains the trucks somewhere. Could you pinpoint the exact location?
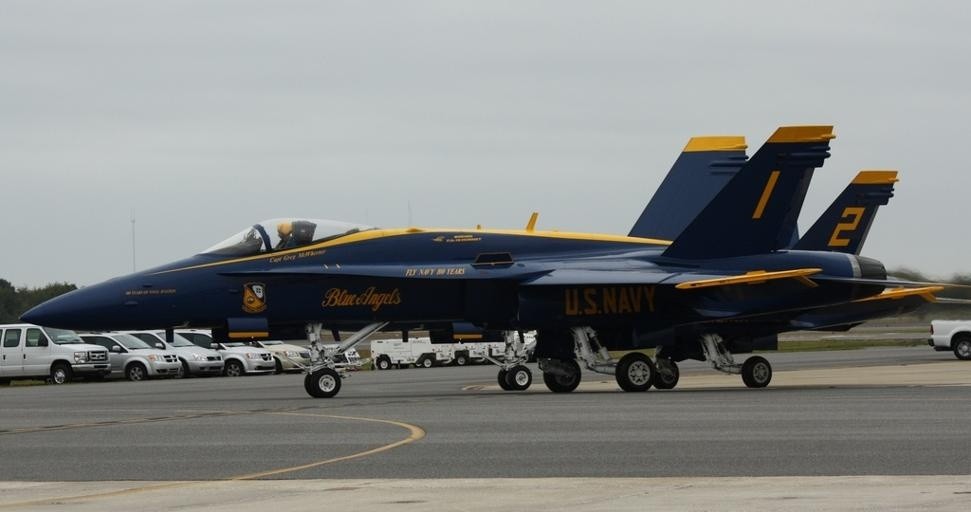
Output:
[369,335,506,370]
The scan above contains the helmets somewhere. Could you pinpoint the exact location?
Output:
[277,222,293,235]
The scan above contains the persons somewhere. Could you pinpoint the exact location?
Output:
[273,222,296,251]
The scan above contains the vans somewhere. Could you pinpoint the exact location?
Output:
[0,323,113,386]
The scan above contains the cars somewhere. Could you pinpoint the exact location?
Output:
[75,329,362,382]
[928,317,970,361]
